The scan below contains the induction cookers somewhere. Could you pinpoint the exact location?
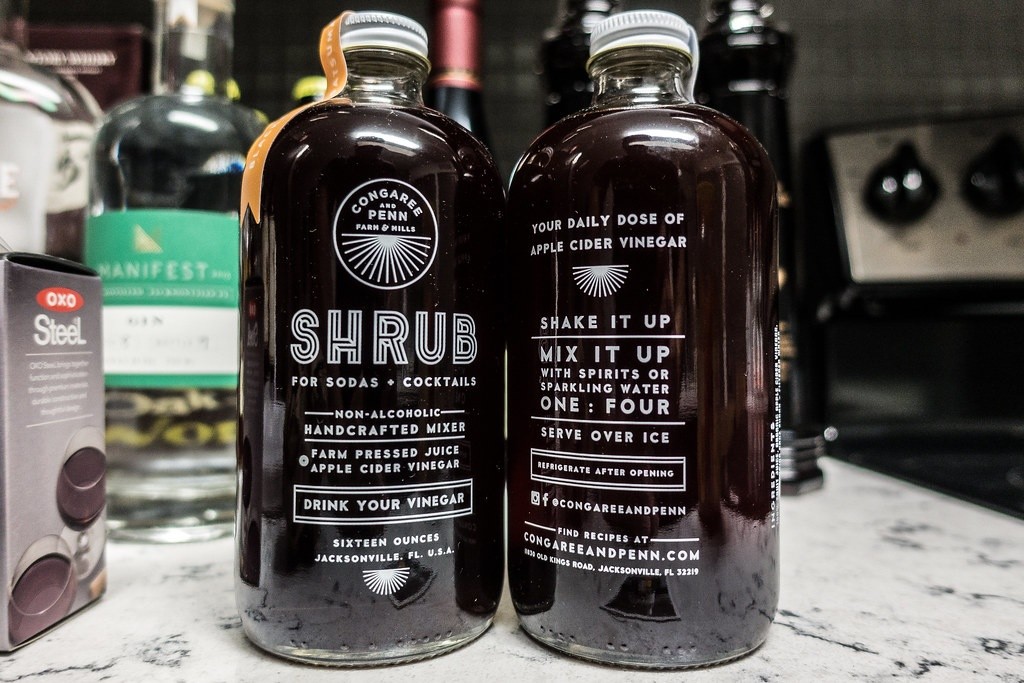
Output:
[798,109,1024,517]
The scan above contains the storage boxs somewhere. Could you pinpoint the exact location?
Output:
[0,252,109,654]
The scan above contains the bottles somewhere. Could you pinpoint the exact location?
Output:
[431,0,491,149]
[292,75,327,108]
[504,10,780,670]
[9,17,102,263]
[238,11,503,668]
[700,0,826,493]
[88,0,266,553]
[539,0,615,135]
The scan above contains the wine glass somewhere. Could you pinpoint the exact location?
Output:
[55,424,107,582]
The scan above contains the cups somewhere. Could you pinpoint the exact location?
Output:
[0,42,66,257]
[9,534,76,646]
